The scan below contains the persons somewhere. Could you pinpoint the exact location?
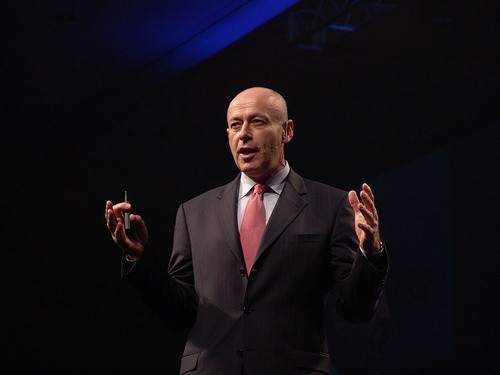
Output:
[103,87,386,375]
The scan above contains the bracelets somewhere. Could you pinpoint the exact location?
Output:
[365,241,383,254]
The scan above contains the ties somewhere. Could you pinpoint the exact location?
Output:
[239,184,267,279]
